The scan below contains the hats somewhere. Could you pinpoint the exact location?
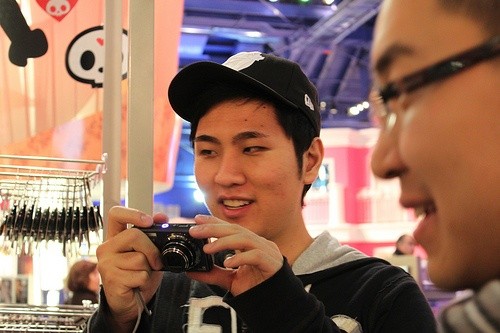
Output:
[168,50,321,136]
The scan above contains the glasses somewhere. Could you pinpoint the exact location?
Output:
[366,38,500,130]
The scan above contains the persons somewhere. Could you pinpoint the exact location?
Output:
[369,0,500,333]
[393,235,417,256]
[84,52,438,333]
[63,260,101,304]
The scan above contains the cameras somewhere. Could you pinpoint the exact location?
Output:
[131,223,214,273]
